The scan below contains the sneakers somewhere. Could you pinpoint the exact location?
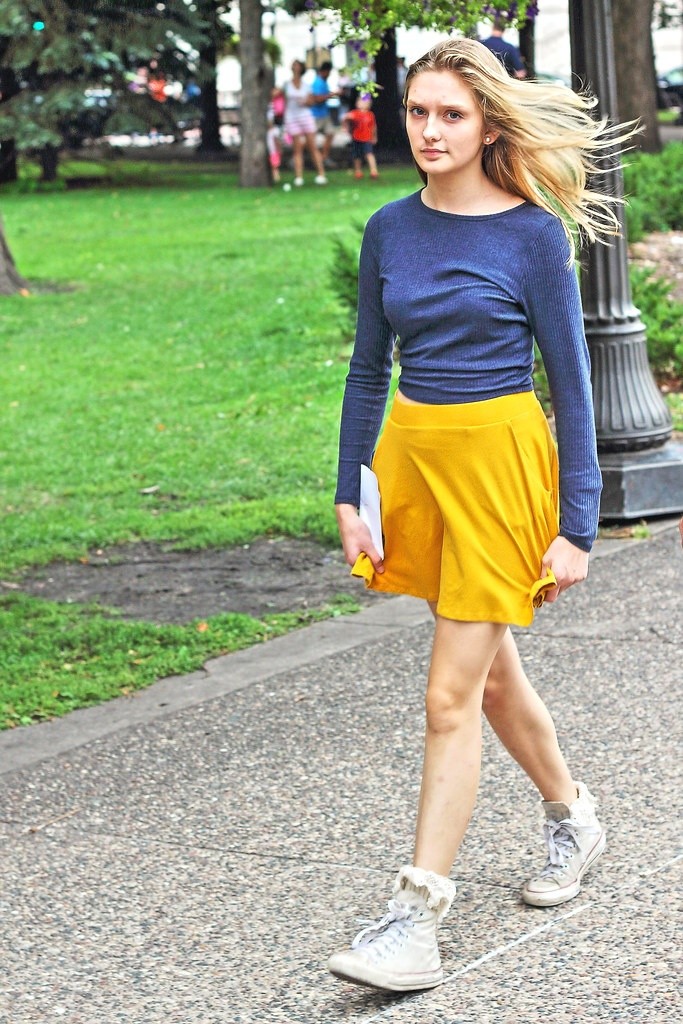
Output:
[328,890,443,991]
[522,781,606,906]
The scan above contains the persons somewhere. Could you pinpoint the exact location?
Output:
[479,13,529,80]
[325,38,609,995]
[265,45,408,188]
[124,54,202,144]
